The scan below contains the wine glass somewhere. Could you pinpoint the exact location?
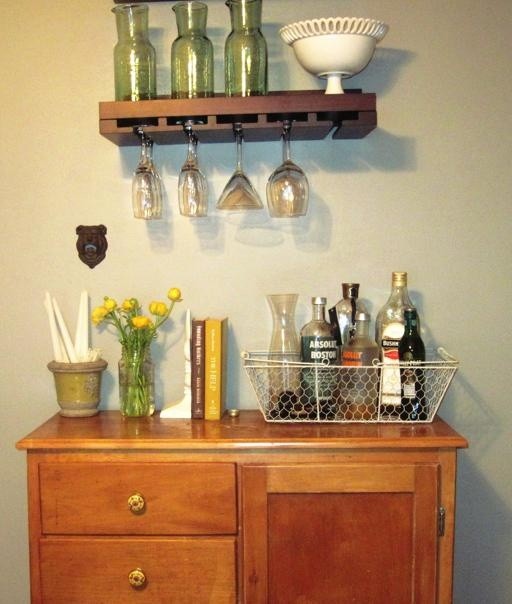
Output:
[279,17,390,94]
[131,115,309,219]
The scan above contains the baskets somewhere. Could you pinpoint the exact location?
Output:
[239,344,461,426]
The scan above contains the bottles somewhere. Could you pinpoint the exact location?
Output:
[268,293,302,406]
[299,270,426,420]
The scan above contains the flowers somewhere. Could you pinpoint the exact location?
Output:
[91,286,185,417]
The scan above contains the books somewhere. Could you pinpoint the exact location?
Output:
[204,315,230,421]
[192,316,210,419]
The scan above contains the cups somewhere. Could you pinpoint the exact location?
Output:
[46,359,108,417]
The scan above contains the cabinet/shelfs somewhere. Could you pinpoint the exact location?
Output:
[236,404,470,603]
[15,406,240,603]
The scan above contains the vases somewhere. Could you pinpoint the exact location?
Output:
[265,290,305,410]
[114,334,158,420]
